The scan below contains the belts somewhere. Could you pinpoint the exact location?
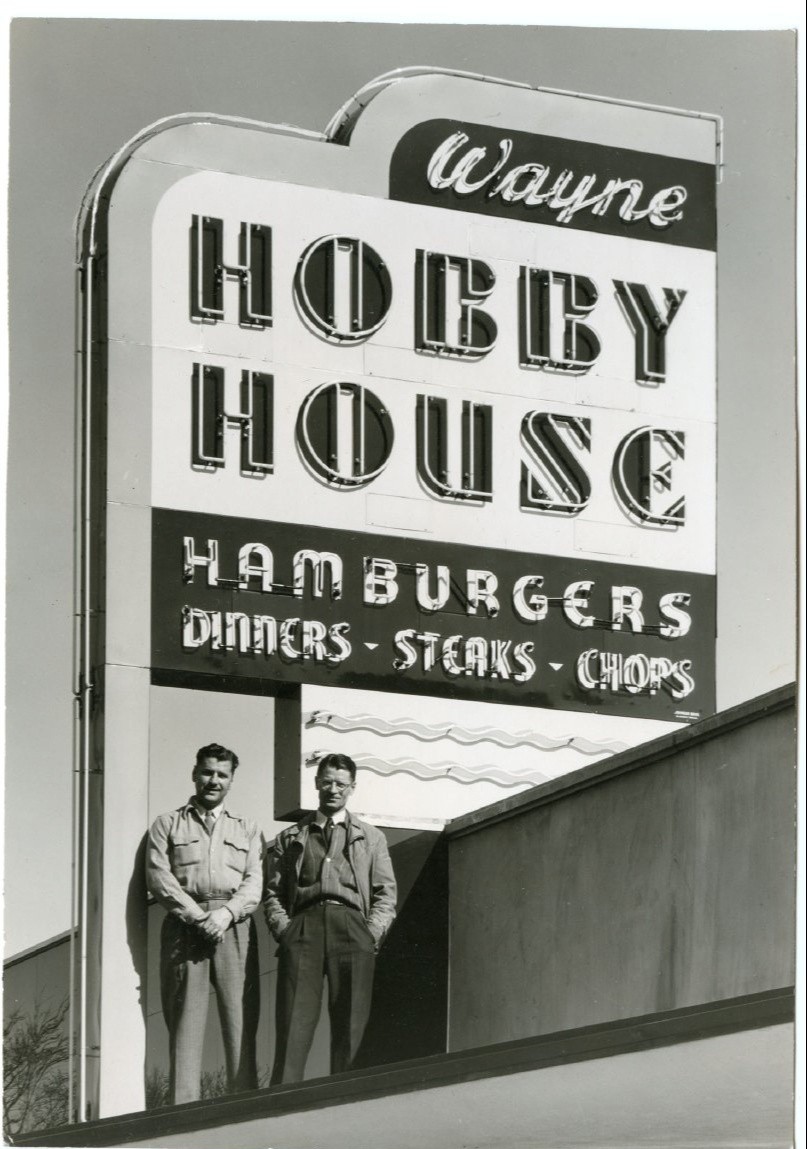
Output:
[190,893,233,901]
[312,898,344,906]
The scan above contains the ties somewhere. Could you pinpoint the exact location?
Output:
[325,818,333,846]
[205,810,215,836]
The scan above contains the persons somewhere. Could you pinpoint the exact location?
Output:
[146,743,267,1105]
[263,754,398,1087]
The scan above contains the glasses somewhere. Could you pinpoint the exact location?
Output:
[318,778,354,791]
